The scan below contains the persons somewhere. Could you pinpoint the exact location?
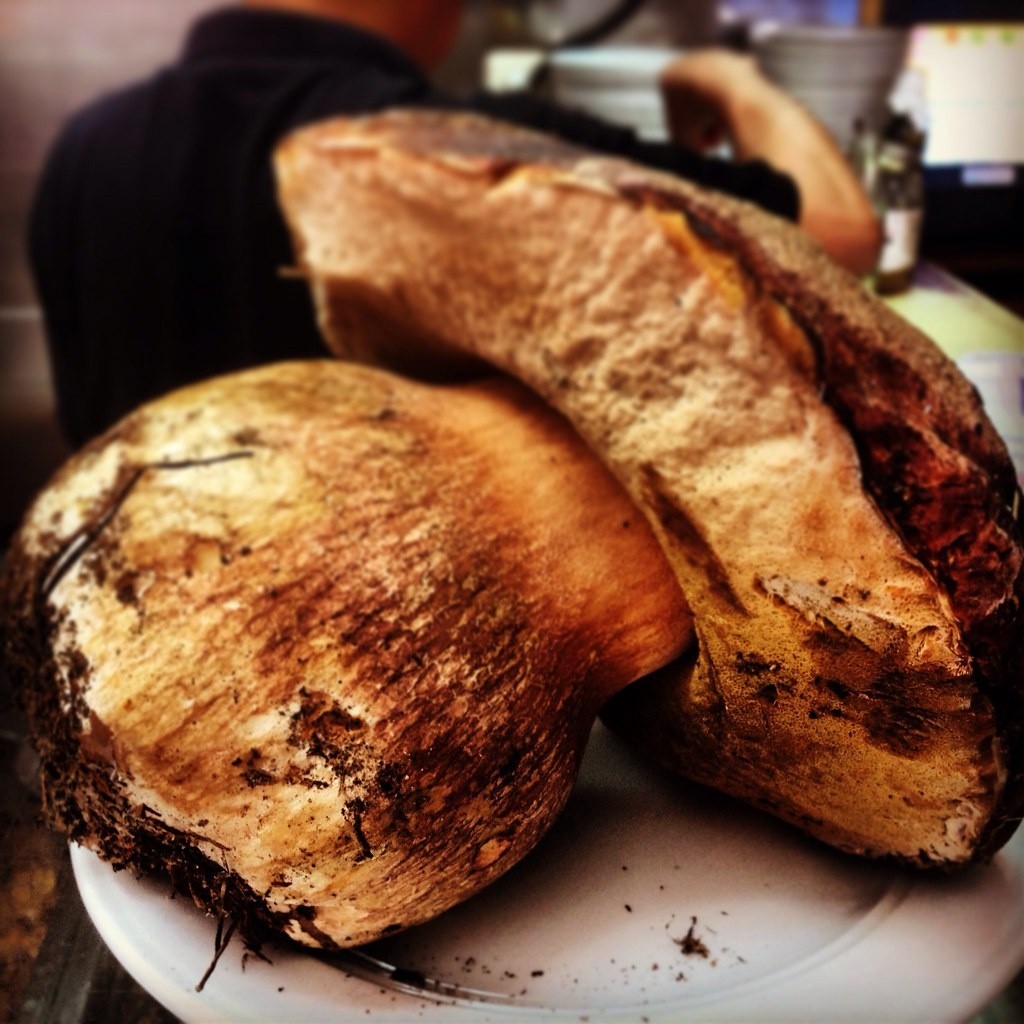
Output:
[31,0,884,457]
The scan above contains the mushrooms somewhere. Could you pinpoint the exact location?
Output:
[0,102,1023,955]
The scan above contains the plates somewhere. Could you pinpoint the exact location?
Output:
[62,274,1022,1024]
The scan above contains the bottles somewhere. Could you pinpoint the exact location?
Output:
[838,121,882,185]
[875,111,925,298]
